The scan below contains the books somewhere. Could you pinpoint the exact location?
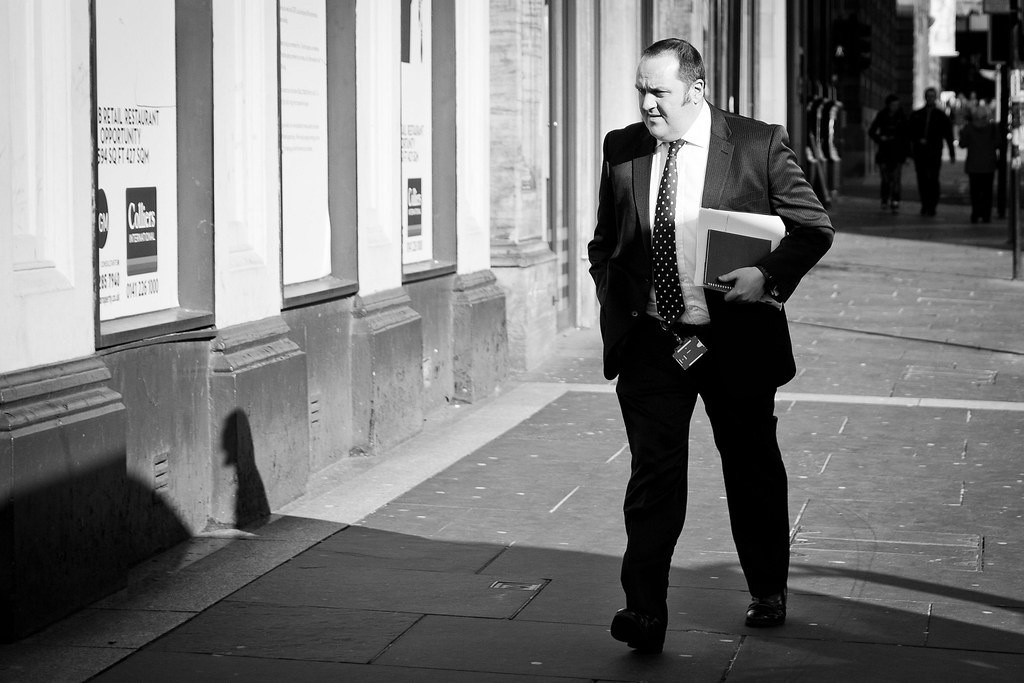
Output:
[704,229,772,294]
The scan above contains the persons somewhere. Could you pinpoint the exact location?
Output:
[868,87,1004,224]
[587,39,835,655]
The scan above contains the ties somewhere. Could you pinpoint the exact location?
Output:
[652,137,687,326]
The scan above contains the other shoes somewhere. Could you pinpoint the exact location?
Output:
[890,201,898,209]
[881,202,887,209]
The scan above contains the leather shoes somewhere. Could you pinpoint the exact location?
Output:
[743,593,787,630]
[609,607,666,654]
